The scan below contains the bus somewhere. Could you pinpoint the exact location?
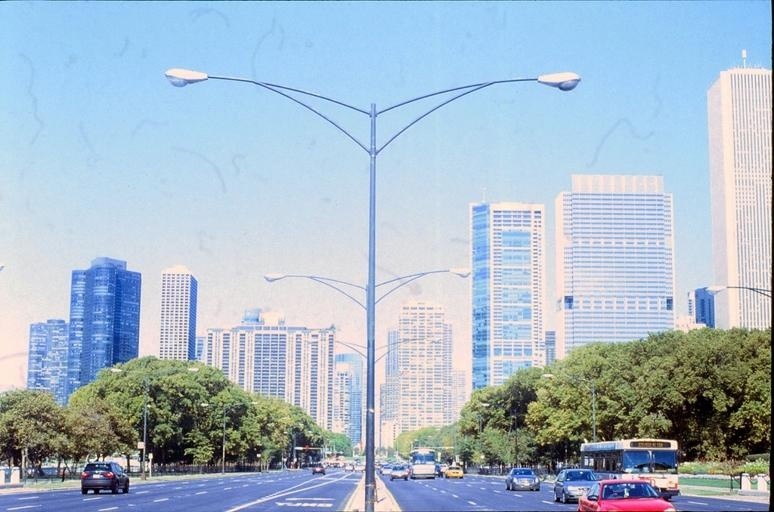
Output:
[579,438,679,501]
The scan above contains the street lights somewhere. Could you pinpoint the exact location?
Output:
[165,68,582,512]
[541,373,595,442]
[111,367,199,480]
[705,285,773,301]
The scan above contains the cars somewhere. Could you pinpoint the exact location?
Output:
[577,479,676,512]
[553,469,596,504]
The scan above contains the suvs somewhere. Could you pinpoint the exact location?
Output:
[81,462,130,494]
